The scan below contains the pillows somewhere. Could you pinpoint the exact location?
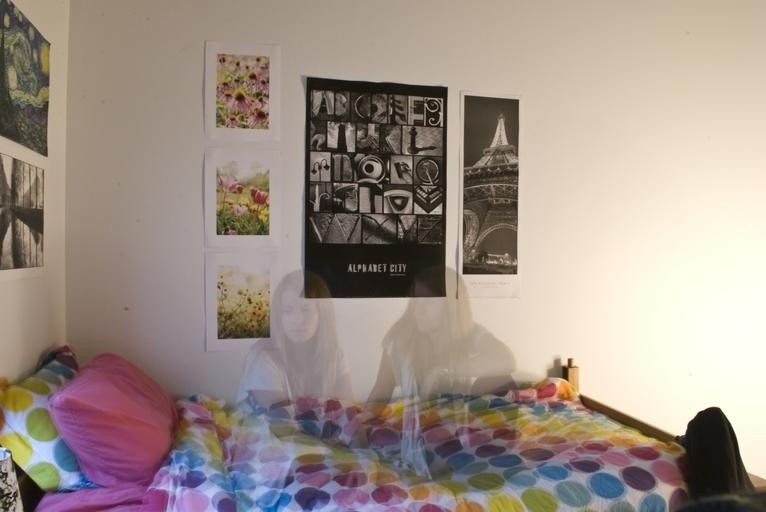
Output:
[0,344,178,494]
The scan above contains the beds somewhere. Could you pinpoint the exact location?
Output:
[18,358,766,512]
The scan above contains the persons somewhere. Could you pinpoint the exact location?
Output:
[227,267,414,512]
[342,262,520,492]
